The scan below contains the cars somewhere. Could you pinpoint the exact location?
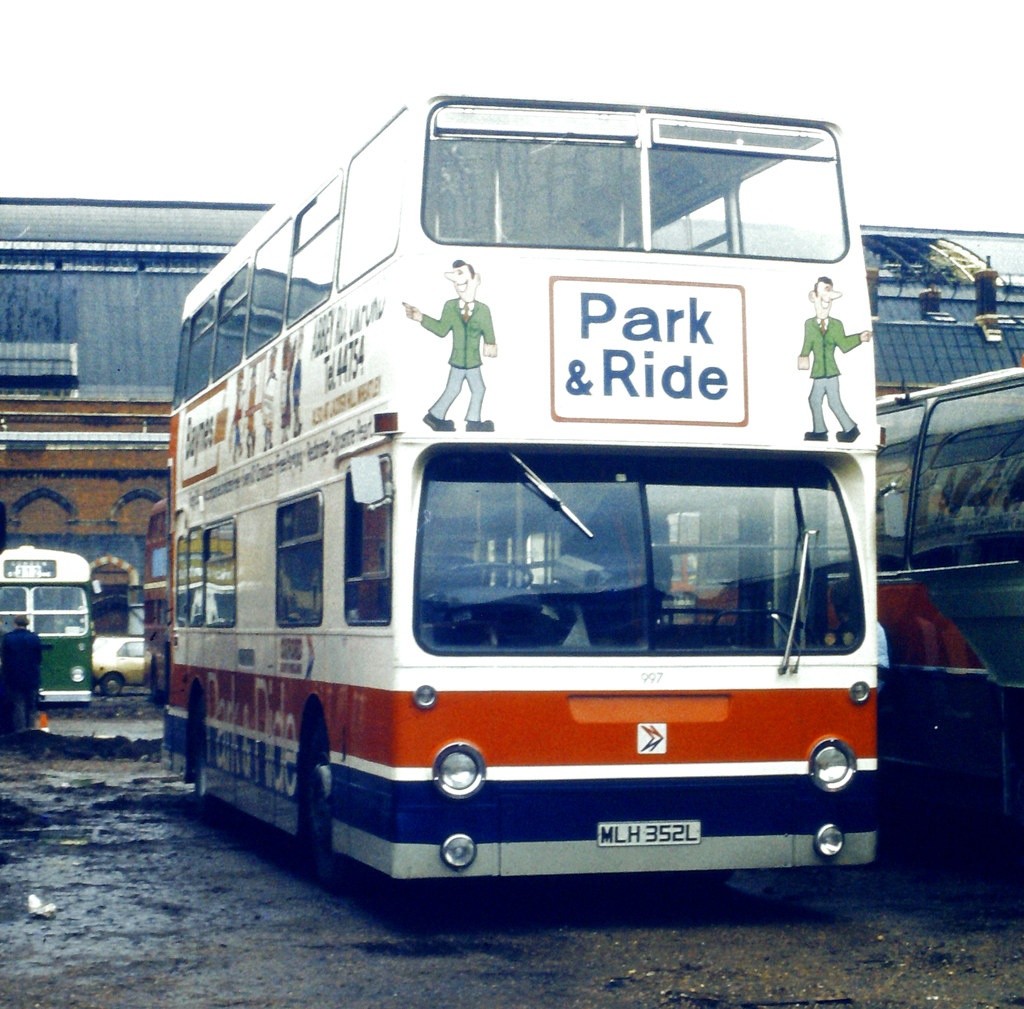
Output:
[91,635,145,698]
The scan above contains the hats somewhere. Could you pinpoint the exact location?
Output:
[15,615,29,625]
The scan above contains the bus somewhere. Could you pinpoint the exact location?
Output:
[826,364,1024,851]
[1,545,103,708]
[156,93,883,884]
[140,496,171,705]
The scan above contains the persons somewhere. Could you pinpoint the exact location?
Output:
[0,616,44,732]
[830,581,890,698]
